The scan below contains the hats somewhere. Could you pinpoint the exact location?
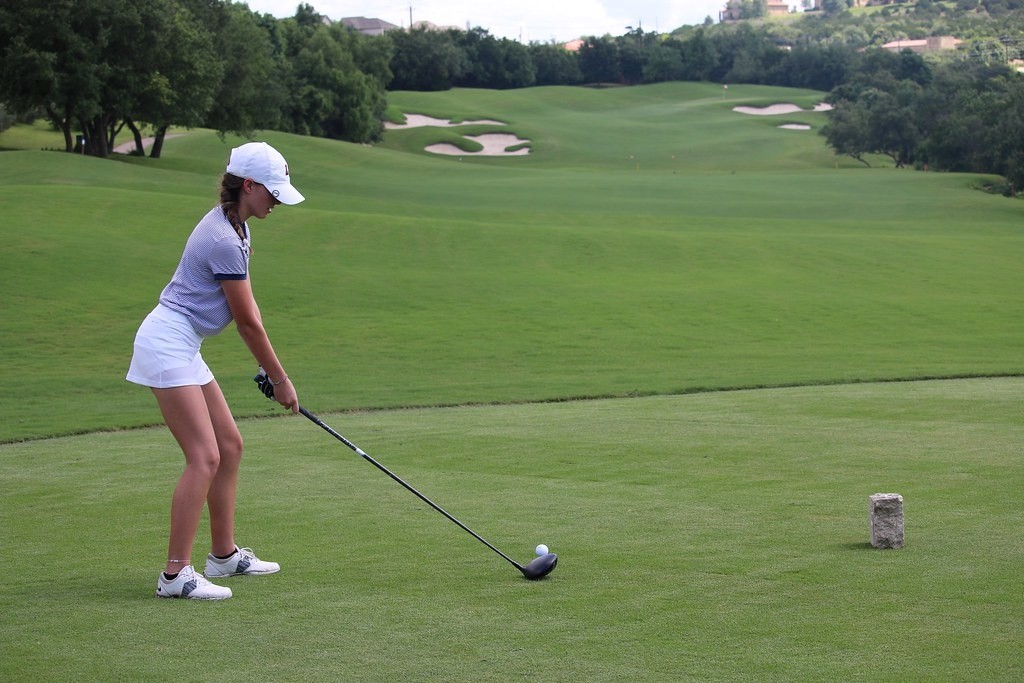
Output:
[226,141,305,206]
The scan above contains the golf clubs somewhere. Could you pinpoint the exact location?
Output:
[254,368,557,585]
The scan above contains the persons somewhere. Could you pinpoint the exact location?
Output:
[125,141,305,601]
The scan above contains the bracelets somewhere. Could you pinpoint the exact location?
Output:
[273,375,288,385]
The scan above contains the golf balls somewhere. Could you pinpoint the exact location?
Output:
[535,543,549,556]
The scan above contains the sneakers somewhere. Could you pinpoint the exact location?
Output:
[156,565,233,600]
[204,544,281,578]
